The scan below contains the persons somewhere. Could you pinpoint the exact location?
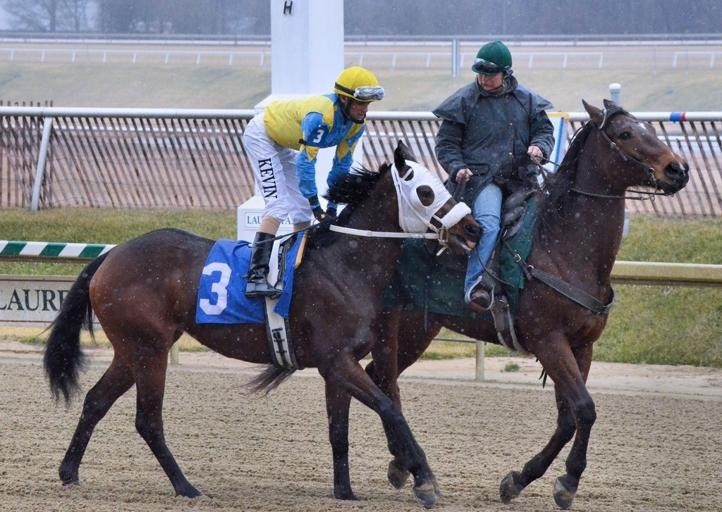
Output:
[240,66,385,299]
[432,39,556,312]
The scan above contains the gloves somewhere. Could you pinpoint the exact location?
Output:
[309,195,338,226]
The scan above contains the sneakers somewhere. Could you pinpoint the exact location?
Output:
[465,289,490,308]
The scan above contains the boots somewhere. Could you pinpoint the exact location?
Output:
[245,231,285,297]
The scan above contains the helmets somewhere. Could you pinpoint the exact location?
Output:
[334,65,384,102]
[472,40,512,75]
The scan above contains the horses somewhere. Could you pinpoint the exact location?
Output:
[27,139,484,510]
[243,97,690,511]
